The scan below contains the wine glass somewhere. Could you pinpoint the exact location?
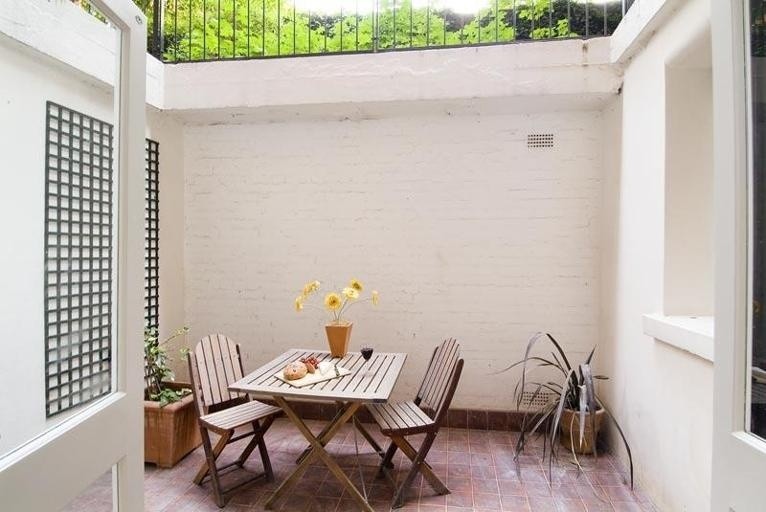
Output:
[359,342,374,371]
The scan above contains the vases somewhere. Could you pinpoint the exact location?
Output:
[324,321,354,358]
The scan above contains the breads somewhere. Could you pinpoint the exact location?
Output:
[307,361,315,374]
[282,361,307,381]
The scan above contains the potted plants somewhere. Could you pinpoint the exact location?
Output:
[487,330,636,502]
[145,326,205,469]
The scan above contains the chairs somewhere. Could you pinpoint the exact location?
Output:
[364,339,464,508]
[189,333,283,507]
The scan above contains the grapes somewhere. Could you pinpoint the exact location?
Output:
[301,357,320,369]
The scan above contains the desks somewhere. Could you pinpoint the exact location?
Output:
[228,349,406,510]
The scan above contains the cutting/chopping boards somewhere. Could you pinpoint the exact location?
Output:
[274,362,352,388]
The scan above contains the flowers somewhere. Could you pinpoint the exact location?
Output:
[294,280,378,323]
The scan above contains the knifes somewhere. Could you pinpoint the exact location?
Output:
[334,363,340,376]
